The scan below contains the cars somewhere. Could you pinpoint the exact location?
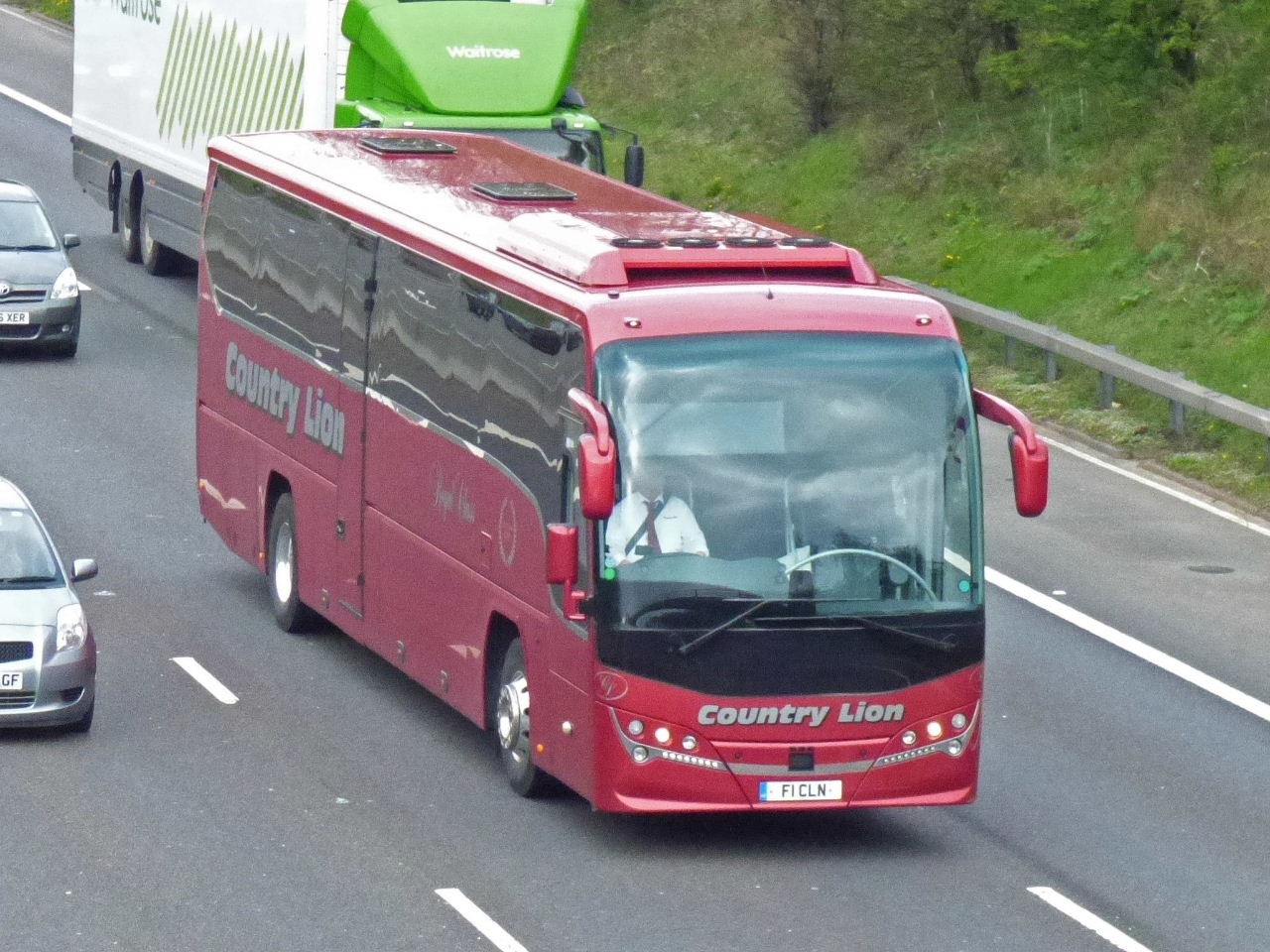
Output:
[1,177,83,362]
[0,474,100,734]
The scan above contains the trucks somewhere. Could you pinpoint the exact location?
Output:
[68,1,648,278]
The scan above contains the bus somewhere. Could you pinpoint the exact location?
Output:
[190,127,1050,821]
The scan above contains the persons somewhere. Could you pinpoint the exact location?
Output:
[605,460,708,566]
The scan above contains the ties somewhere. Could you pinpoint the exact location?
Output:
[645,502,661,554]
